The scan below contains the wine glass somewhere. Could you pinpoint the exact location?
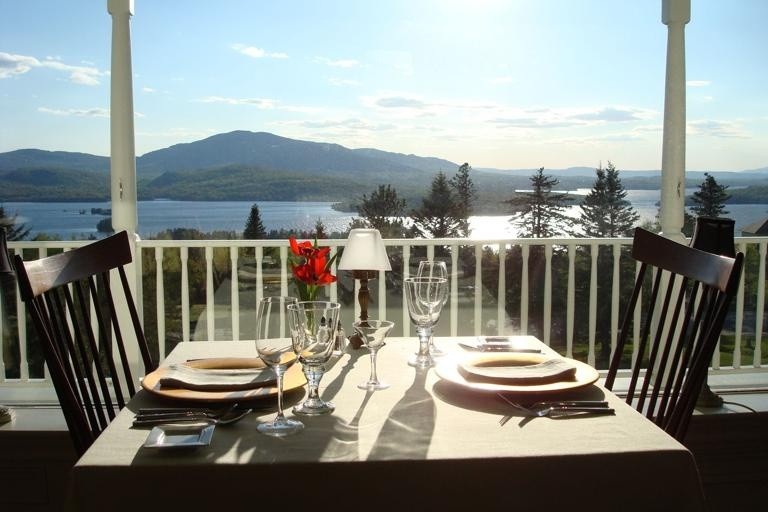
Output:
[255,296,304,437]
[412,260,447,357]
[286,300,341,416]
[404,276,448,369]
[350,317,395,391]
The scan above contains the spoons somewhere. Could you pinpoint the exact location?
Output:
[132,403,252,427]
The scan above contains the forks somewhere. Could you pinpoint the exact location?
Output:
[497,393,615,416]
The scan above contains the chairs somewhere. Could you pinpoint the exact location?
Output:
[603,226,744,446]
[14,229,158,460]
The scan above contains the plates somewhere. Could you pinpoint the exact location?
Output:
[436,353,601,397]
[142,420,217,450]
[142,357,312,406]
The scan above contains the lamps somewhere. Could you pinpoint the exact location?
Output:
[686,217,735,409]
[337,228,393,350]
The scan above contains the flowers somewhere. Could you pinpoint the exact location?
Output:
[286,236,338,332]
